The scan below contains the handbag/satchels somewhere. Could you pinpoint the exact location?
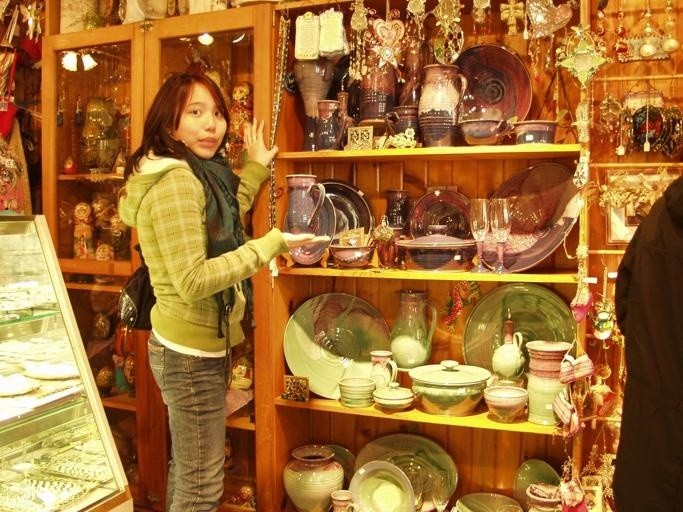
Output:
[119,245,156,330]
[560,339,595,384]
[553,384,579,439]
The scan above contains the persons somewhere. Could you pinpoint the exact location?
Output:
[611,175,682,511]
[115,69,332,511]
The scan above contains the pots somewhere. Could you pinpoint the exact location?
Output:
[396,224,476,272]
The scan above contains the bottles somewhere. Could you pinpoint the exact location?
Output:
[384,190,409,239]
[525,485,561,512]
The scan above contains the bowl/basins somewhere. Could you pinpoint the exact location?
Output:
[457,119,508,145]
[329,246,377,268]
[512,120,558,144]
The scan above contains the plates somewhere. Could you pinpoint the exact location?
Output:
[322,182,372,245]
[283,189,336,266]
[352,433,459,512]
[512,459,561,512]
[455,492,523,512]
[474,162,582,273]
[327,445,355,489]
[348,460,415,512]
[455,47,533,127]
[282,292,391,400]
[461,282,575,376]
[409,190,474,240]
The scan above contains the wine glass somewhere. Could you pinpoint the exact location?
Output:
[489,196,512,274]
[407,471,424,512]
[471,199,487,274]
[431,470,450,512]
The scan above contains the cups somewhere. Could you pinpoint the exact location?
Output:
[331,490,359,512]
[483,386,528,423]
[376,227,407,271]
[338,377,376,407]
